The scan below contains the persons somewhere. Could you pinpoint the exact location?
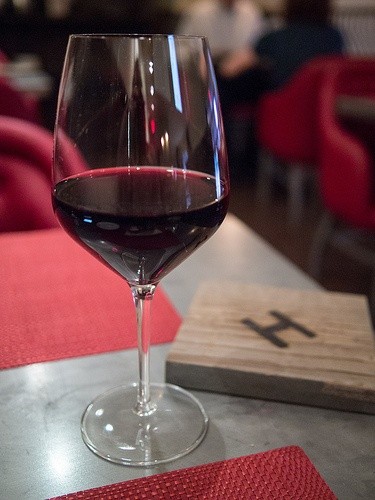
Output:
[170,1,344,184]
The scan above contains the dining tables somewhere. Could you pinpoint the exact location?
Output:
[1,212,374,499]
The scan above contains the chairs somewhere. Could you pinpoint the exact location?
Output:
[308,59,374,265]
[0,115,92,232]
[256,55,352,218]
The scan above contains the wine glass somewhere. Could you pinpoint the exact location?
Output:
[51,30,230,467]
